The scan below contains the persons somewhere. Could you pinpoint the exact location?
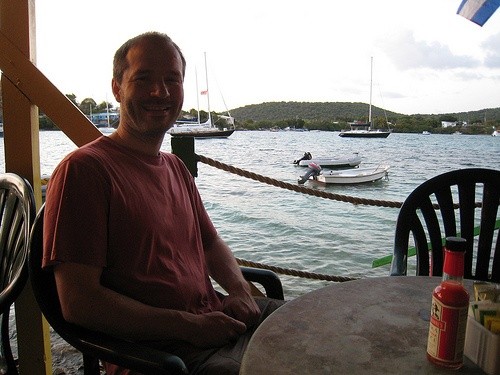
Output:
[39,30,288,375]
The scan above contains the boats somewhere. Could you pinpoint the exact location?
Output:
[293,152,362,169]
[297,162,391,185]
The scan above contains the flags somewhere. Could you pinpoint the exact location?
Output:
[456,0,500,27]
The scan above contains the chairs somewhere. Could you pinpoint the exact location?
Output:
[0,172,284,375]
[390,168,500,283]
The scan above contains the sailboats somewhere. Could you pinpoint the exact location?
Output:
[338,56,391,138]
[166,50,236,139]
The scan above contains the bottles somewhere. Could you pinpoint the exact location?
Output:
[426,237,469,370]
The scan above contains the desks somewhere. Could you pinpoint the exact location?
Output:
[240,275,500,375]
